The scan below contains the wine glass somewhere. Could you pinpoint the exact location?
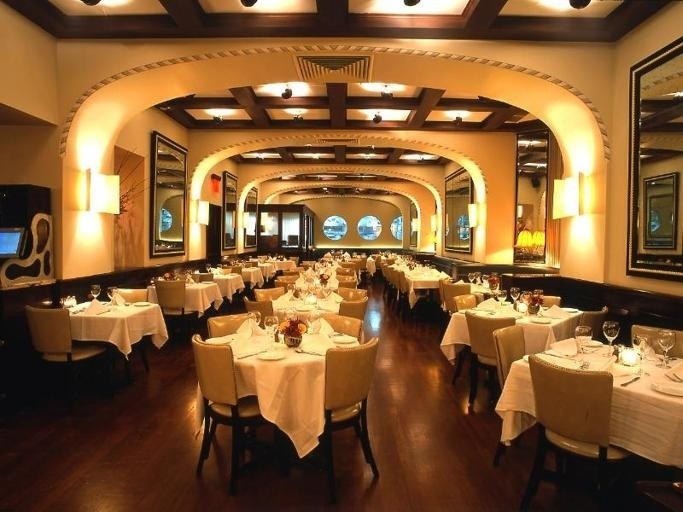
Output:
[90,285,117,305]
[154,250,449,283]
[468,271,548,313]
[90,302,151,316]
[573,321,683,399]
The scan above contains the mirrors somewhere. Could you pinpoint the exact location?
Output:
[444,166,474,254]
[243,187,260,249]
[642,171,678,251]
[148,130,188,259]
[220,169,238,251]
[624,35,683,283]
[512,130,552,264]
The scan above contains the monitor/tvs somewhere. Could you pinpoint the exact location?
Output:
[0,227,26,258]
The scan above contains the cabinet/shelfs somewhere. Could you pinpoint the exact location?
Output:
[0,183,50,280]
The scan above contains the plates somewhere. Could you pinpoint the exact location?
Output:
[459,304,578,324]
[206,287,359,361]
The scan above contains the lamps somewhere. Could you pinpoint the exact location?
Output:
[430,214,438,230]
[241,0,258,7]
[569,0,591,10]
[531,177,541,188]
[553,176,579,222]
[212,84,470,129]
[80,0,101,7]
[198,200,210,226]
[403,0,421,6]
[96,173,122,216]
[514,226,545,260]
[411,217,420,233]
[467,202,479,229]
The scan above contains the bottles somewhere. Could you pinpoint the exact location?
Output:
[64,296,77,307]
